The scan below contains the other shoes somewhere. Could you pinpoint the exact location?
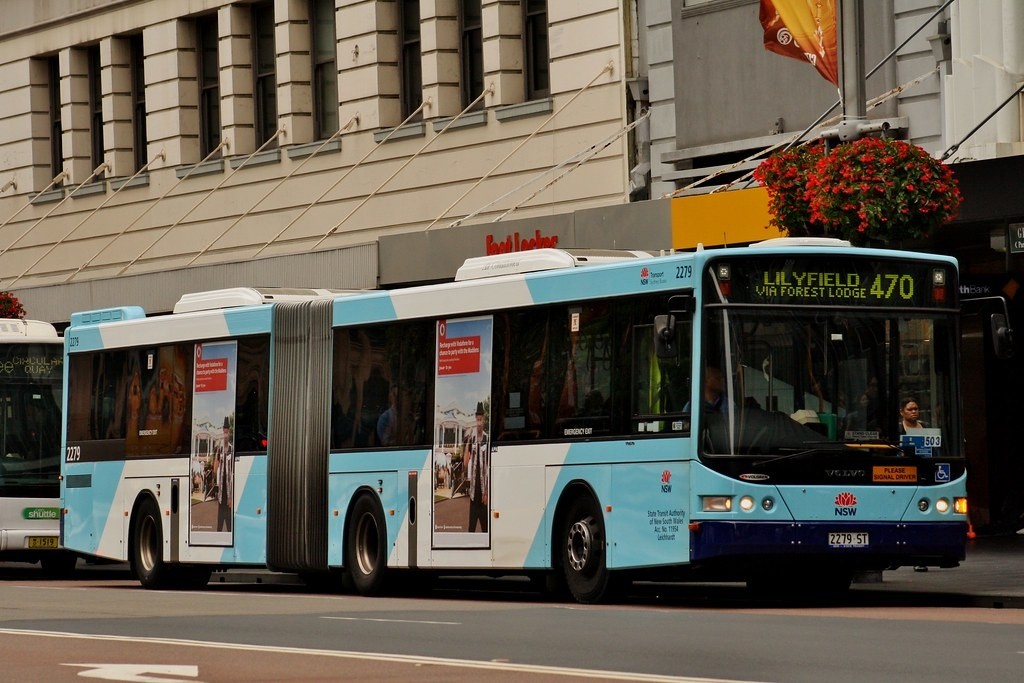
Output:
[914,565,928,572]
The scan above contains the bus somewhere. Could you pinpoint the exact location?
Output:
[59,235,1017,603]
[0,313,78,580]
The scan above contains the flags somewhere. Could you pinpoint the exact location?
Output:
[759,0,839,90]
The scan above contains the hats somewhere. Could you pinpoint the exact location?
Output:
[475,401,485,415]
[223,416,230,428]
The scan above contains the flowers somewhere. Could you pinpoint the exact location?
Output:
[753,136,963,232]
[0,291,27,320]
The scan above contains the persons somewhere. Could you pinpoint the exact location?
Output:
[213,416,233,532]
[463,401,489,532]
[844,375,886,438]
[682,366,736,413]
[377,385,422,446]
[898,397,939,457]
